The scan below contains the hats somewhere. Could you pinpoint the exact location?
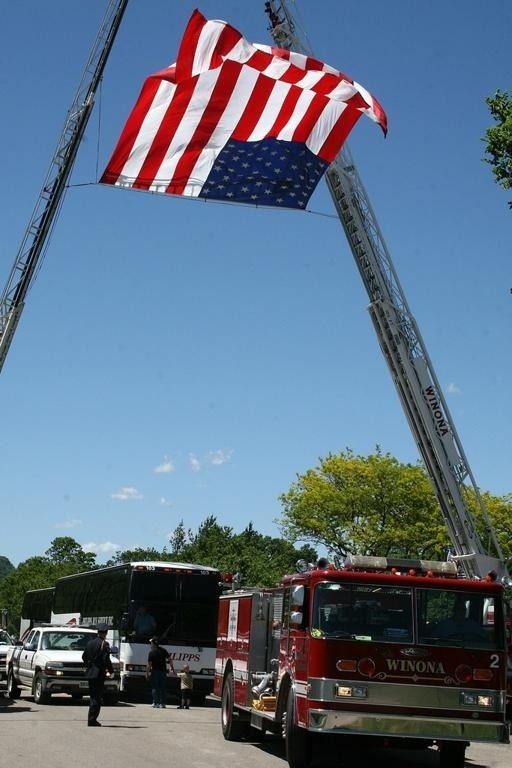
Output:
[180,664,190,673]
[149,636,160,644]
[97,622,109,634]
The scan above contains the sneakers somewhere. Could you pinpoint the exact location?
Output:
[151,703,190,709]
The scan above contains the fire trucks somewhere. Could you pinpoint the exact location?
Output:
[205,556,509,768]
[258,0,510,735]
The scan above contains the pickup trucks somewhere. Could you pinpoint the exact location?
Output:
[5,625,122,706]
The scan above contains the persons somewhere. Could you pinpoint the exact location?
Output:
[144,636,174,708]
[171,663,194,710]
[80,626,115,728]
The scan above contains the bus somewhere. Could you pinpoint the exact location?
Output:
[19,586,57,643]
[51,560,223,703]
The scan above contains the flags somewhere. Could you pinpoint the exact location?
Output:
[94,6,391,215]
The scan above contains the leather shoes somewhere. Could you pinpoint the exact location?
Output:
[88,723,101,726]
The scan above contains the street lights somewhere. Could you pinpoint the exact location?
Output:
[2,607,9,626]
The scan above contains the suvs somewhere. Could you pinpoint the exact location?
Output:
[1,628,16,682]
